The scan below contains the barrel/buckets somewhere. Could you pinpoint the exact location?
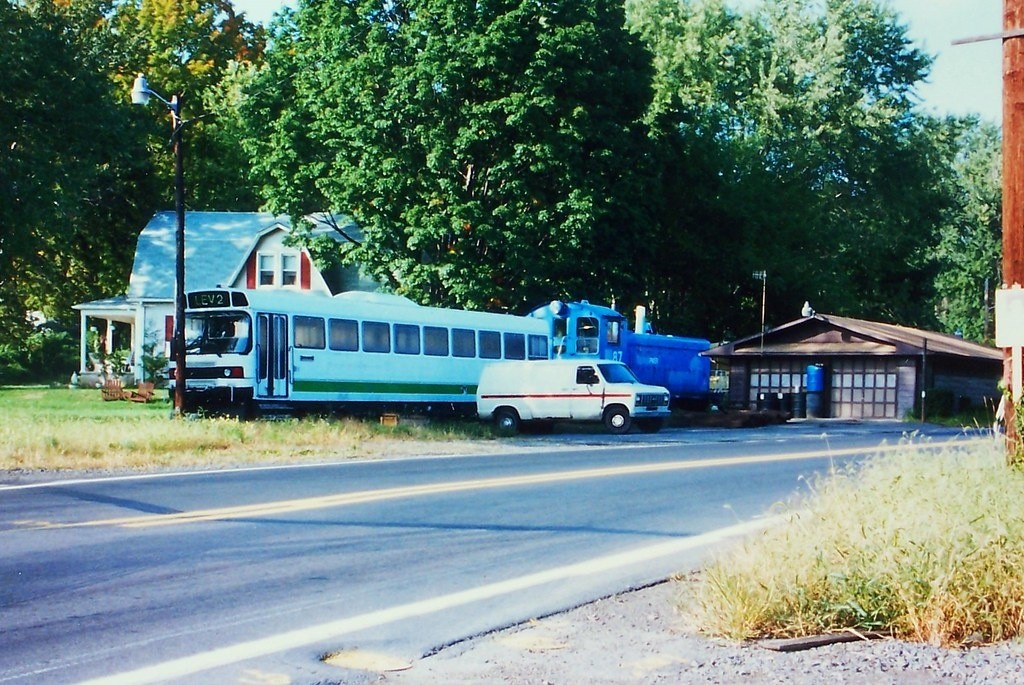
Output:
[789,392,806,418]
[805,364,825,392]
[773,393,789,413]
[807,391,829,417]
[756,392,774,411]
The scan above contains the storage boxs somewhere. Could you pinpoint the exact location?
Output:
[756,364,826,419]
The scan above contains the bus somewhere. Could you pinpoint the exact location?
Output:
[169,285,555,421]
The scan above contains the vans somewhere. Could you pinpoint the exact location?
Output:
[476,358,672,437]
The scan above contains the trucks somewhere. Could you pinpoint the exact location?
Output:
[522,298,714,409]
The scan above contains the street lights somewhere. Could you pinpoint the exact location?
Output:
[131,72,191,419]
